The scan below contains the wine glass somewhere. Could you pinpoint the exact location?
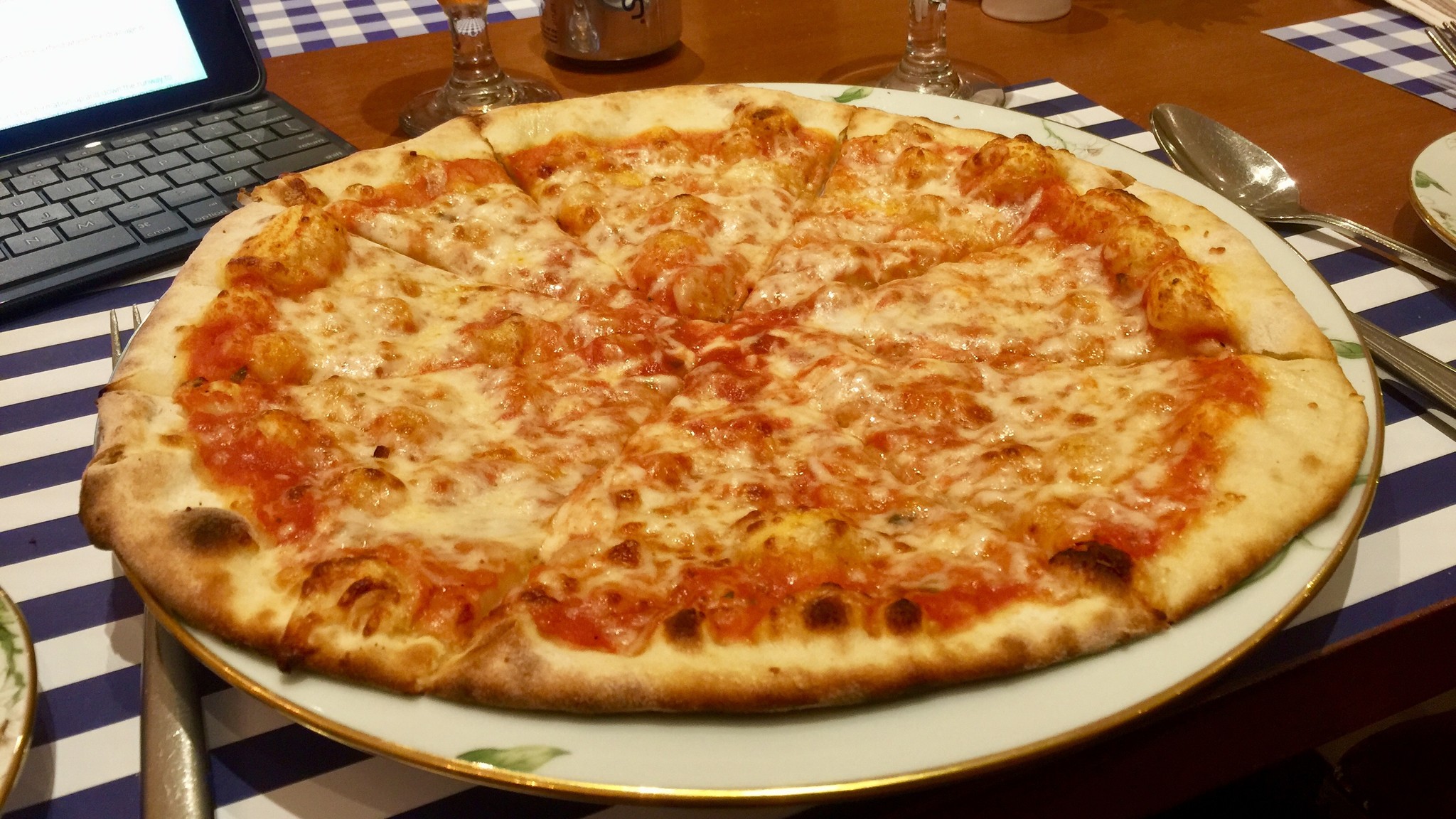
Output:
[394,0,563,139]
[814,0,1005,108]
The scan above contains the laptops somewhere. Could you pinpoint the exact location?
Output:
[0,0,359,312]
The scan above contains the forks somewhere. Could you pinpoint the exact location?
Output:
[108,297,219,819]
[1424,21,1456,72]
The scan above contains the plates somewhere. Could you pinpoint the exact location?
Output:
[0,582,39,810]
[113,81,1383,796]
[1406,130,1456,252]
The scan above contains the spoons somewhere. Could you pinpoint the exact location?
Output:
[1149,101,1456,286]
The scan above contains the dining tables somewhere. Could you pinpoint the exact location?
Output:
[0,0,1456,819]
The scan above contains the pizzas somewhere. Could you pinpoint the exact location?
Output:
[77,82,1372,712]
